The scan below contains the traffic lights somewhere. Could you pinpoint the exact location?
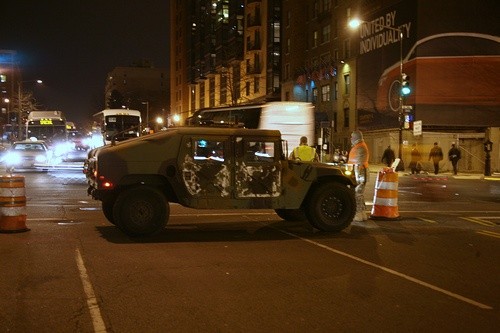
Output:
[401,74,411,96]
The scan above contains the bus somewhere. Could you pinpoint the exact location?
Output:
[90,108,142,148]
[24,110,78,161]
[184,100,316,160]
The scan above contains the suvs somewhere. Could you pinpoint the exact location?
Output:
[81,108,358,239]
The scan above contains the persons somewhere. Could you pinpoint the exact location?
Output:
[347,130,369,221]
[410,144,421,175]
[449,142,461,175]
[429,142,443,175]
[382,145,395,167]
[290,136,319,163]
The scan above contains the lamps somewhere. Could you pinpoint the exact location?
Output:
[483,139,493,177]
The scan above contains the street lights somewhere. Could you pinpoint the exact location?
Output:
[349,19,405,173]
[4,99,10,124]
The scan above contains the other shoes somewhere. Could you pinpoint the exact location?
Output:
[351,212,368,222]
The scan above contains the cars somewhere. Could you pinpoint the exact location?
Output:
[61,134,100,163]
[6,137,51,173]
